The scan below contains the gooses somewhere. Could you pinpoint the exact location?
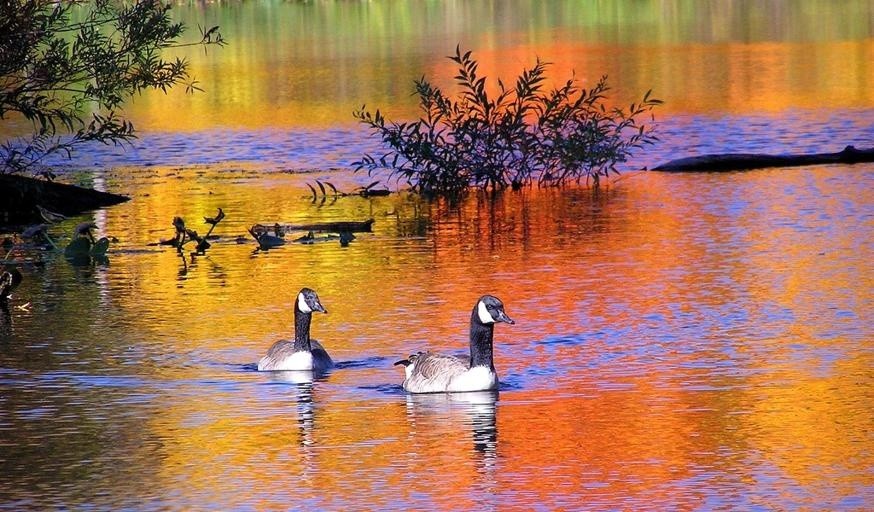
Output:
[257,287,336,373]
[392,294,516,395]
